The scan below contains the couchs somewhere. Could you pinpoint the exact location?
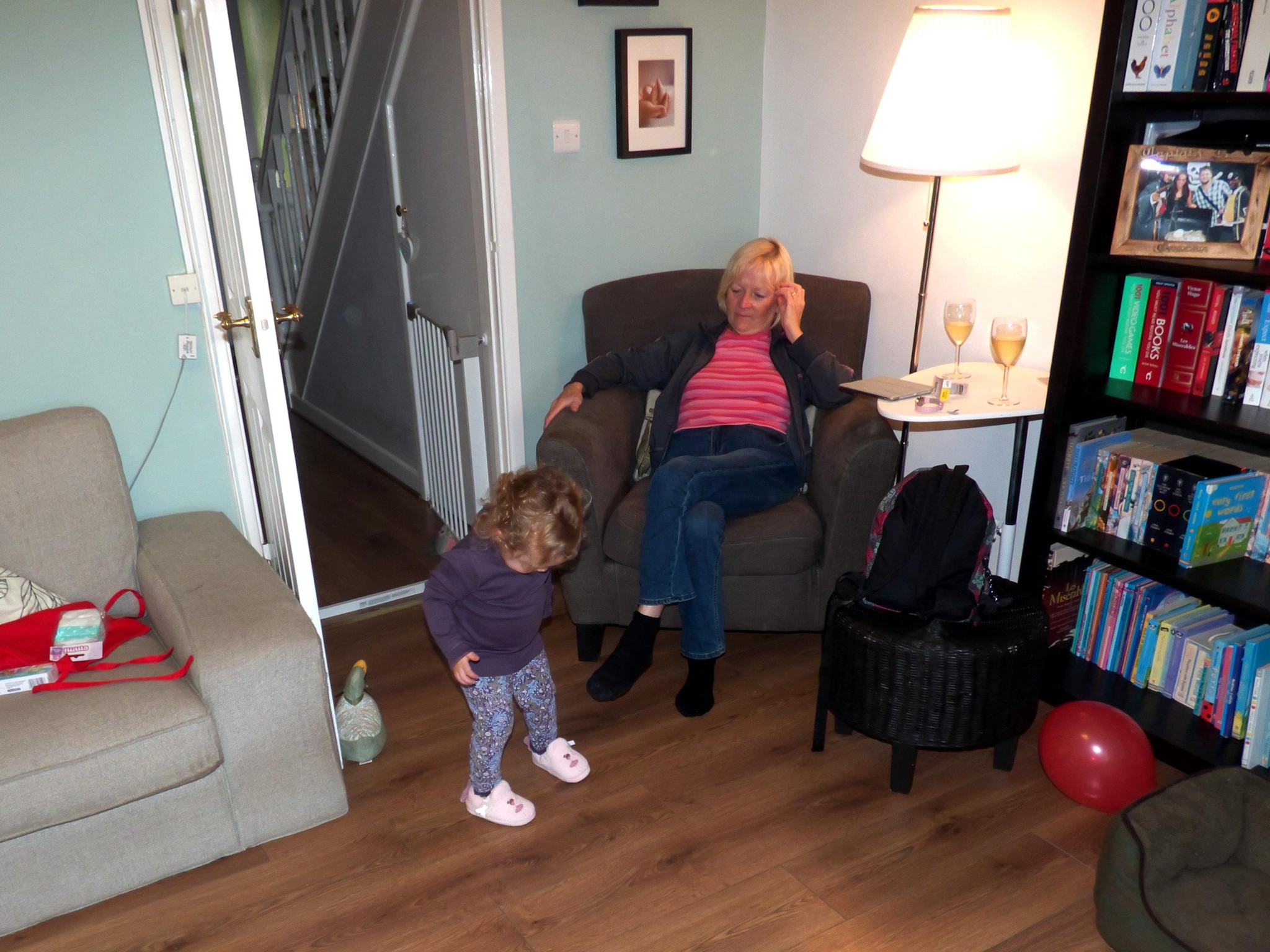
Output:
[531,265,909,671]
[0,398,352,942]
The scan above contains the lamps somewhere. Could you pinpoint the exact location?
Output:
[854,3,1026,488]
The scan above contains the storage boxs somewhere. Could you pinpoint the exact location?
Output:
[0,661,60,696]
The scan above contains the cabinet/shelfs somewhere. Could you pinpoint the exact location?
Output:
[1022,0,1270,773]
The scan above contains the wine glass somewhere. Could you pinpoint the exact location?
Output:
[942,297,977,379]
[987,317,1028,406]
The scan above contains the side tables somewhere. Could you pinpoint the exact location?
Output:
[874,357,1046,581]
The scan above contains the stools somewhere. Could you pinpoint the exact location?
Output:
[807,569,1054,800]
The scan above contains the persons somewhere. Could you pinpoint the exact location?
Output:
[1130,166,1250,242]
[423,465,592,826]
[543,238,859,720]
[639,85,671,127]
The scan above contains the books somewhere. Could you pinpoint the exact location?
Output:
[1109,273,1270,409]
[1123,0,1270,92]
[1055,415,1270,568]
[1042,543,1270,770]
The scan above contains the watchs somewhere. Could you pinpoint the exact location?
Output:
[915,396,944,413]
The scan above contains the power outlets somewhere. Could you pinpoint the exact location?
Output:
[166,270,202,307]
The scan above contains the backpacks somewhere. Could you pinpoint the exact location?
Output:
[865,464,997,625]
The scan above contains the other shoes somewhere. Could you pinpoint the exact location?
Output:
[523,733,591,783]
[460,775,536,827]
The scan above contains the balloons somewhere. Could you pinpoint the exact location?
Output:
[1038,700,1156,813]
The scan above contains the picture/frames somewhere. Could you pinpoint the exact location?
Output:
[612,24,694,162]
[1107,139,1270,263]
